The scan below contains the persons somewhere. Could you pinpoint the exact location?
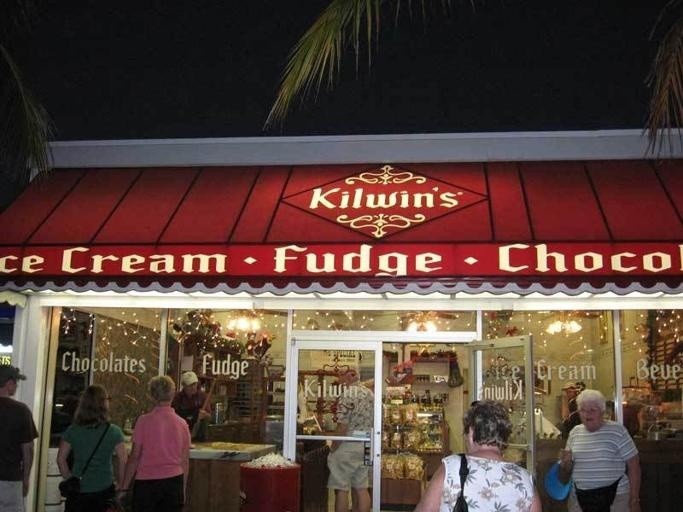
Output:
[0,364,42,512]
[411,398,543,512]
[557,388,643,512]
[56,384,129,512]
[323,363,375,512]
[115,374,191,511]
[170,369,213,443]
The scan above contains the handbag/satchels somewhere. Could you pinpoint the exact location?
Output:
[59,475,81,497]
[544,458,572,501]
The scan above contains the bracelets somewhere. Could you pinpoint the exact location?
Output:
[629,498,641,504]
[120,489,129,494]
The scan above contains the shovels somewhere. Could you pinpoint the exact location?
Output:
[191,377,217,438]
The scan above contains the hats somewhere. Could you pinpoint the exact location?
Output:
[180,372,198,386]
[0,365,26,382]
[561,381,574,389]
